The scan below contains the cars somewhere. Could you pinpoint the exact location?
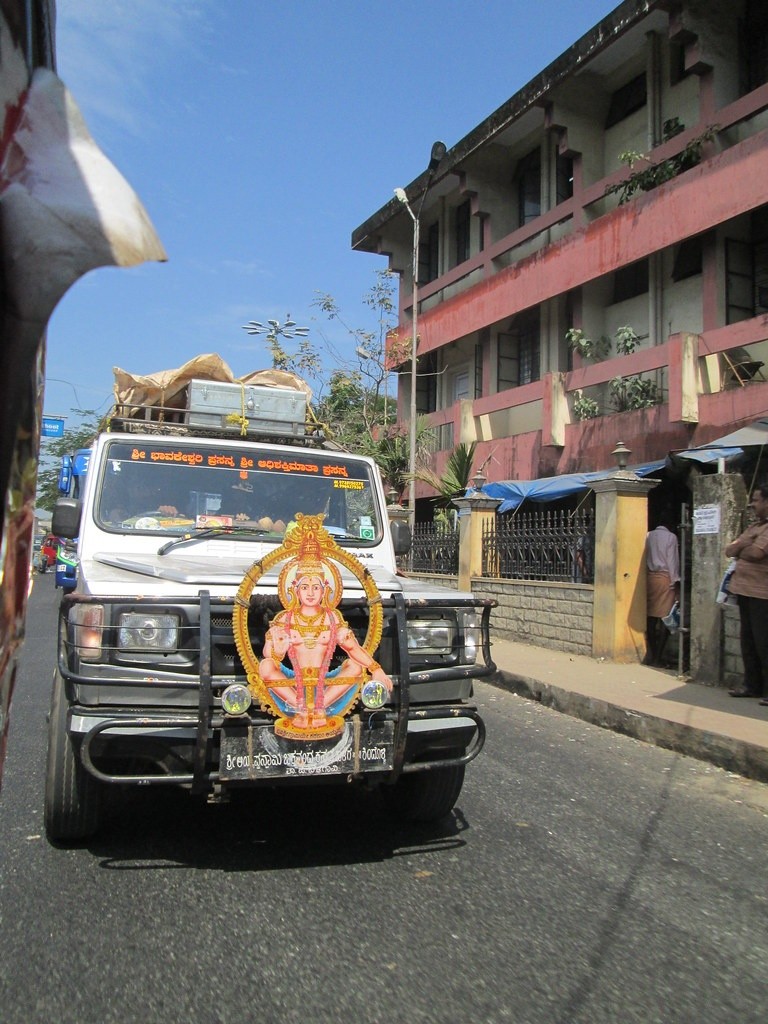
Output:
[32,544,44,569]
[32,533,47,545]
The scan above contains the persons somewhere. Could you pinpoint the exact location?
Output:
[110,477,177,519]
[725,482,768,705]
[641,509,681,669]
[576,513,595,584]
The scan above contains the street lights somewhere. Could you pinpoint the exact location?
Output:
[394,188,419,566]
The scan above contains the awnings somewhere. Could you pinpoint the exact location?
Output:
[456,415,768,514]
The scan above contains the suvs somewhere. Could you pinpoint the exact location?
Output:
[45,434,479,842]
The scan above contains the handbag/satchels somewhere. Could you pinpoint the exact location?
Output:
[715,560,739,607]
[662,602,680,636]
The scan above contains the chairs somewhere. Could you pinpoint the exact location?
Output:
[721,347,765,387]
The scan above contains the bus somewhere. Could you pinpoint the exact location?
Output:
[53,448,97,596]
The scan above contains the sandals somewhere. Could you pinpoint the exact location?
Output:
[759,697,768,705]
[728,685,760,696]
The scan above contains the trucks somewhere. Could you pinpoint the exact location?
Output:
[41,535,61,567]
